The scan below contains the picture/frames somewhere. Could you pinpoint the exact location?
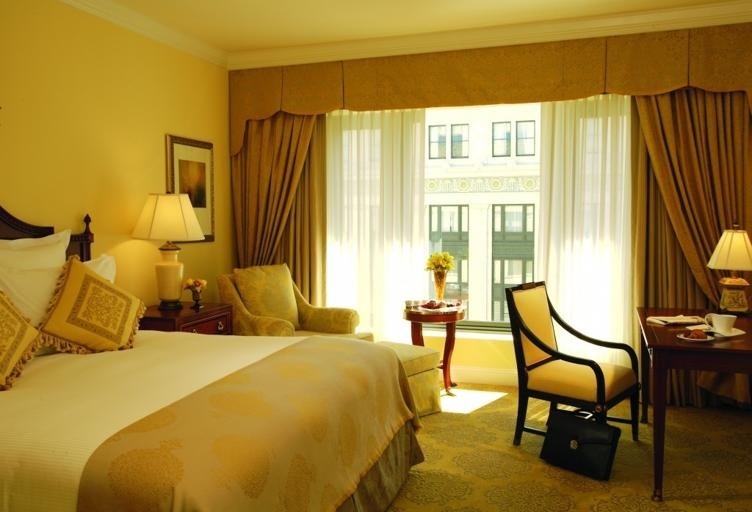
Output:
[164,132,216,245]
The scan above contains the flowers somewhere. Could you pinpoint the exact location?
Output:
[422,251,454,272]
[183,277,207,295]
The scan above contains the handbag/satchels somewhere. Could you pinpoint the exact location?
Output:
[538,406,622,481]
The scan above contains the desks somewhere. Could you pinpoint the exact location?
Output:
[632,299,752,503]
[403,301,465,396]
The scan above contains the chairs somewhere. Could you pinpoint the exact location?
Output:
[499,278,645,449]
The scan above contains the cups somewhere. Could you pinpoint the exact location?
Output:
[405,299,426,308]
[704,313,737,334]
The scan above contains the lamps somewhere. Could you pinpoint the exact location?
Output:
[129,190,205,313]
[704,222,751,318]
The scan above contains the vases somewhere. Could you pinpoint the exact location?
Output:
[430,268,448,302]
[190,288,205,311]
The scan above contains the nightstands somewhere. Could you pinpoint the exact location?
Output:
[138,300,234,335]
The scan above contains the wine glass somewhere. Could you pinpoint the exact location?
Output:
[190,287,205,309]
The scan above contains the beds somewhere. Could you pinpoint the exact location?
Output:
[0,202,427,511]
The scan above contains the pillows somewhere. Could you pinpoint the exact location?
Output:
[0,228,73,275]
[231,262,303,332]
[0,268,44,327]
[0,289,43,394]
[0,253,117,331]
[30,251,147,354]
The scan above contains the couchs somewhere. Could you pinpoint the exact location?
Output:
[372,336,444,422]
[216,271,378,343]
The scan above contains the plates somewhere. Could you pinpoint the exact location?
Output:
[685,324,746,339]
[675,333,714,341]
